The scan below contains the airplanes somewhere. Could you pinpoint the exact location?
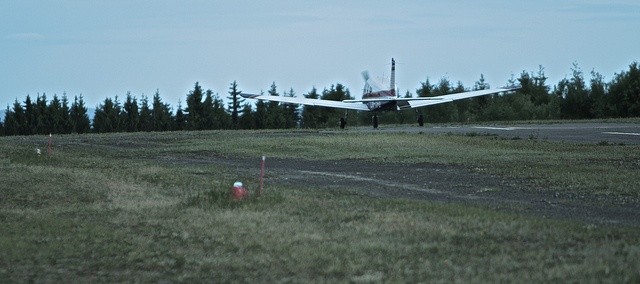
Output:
[240,58,522,129]
